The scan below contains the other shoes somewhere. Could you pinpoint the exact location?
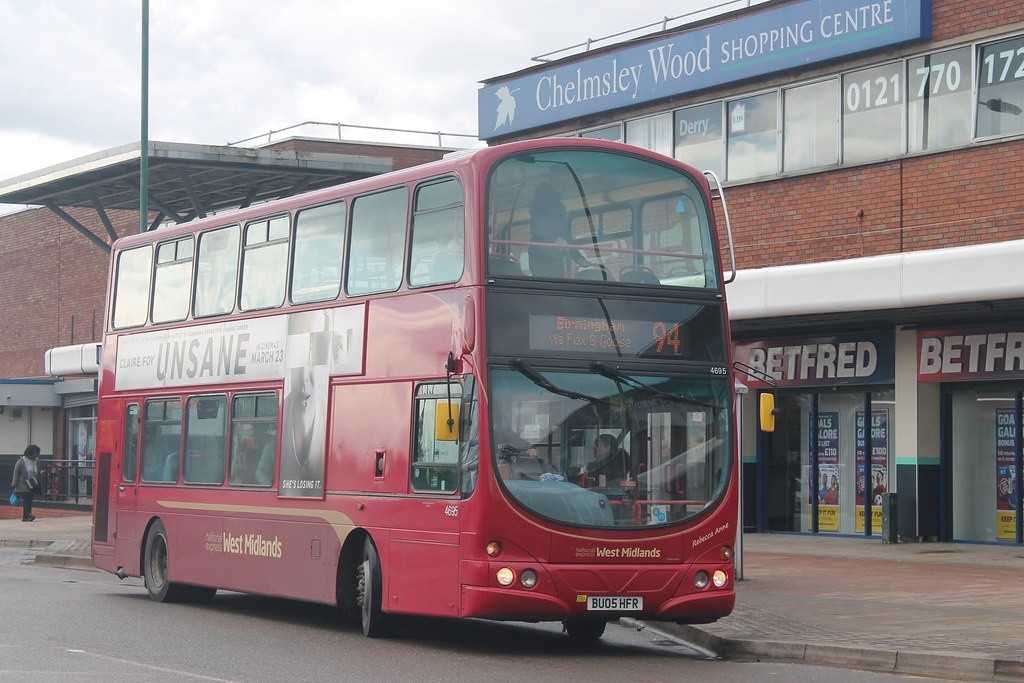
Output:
[22,514,35,523]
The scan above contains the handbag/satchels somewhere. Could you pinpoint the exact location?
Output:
[10,488,20,505]
[25,476,39,491]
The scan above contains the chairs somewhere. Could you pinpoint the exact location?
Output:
[488,254,660,283]
[164,451,181,483]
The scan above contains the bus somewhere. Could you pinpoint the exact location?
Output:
[90,136,783,644]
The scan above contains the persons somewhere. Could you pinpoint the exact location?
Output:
[587,434,629,487]
[493,420,539,457]
[281,311,353,495]
[873,470,887,506]
[11,445,41,521]
[819,473,838,505]
[254,439,275,485]
[528,163,590,277]
